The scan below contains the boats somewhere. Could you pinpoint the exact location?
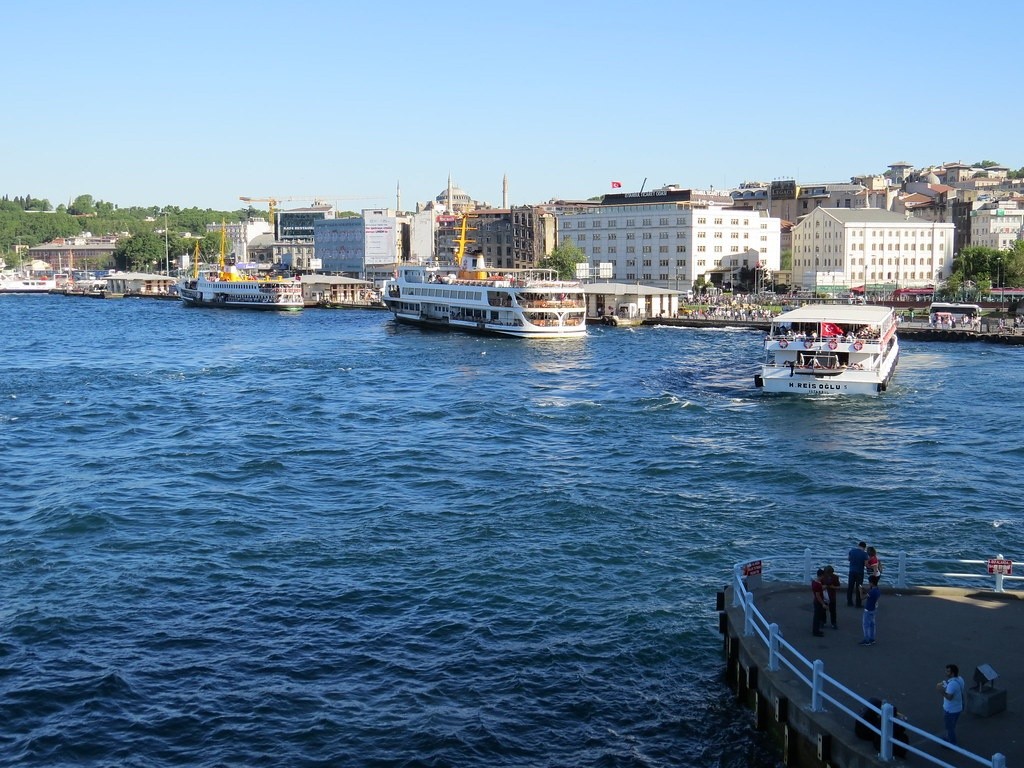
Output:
[380,258,588,340]
[176,264,305,312]
[102,290,125,299]
[755,301,902,398]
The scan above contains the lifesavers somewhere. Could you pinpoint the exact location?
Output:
[804,339,813,349]
[542,302,547,307]
[829,340,838,350]
[540,320,545,326]
[899,329,1008,344]
[854,342,863,350]
[778,339,788,348]
[602,317,617,327]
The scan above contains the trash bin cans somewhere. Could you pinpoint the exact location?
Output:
[966,663,1008,718]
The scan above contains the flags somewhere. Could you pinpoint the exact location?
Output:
[822,323,843,336]
[611,181,621,189]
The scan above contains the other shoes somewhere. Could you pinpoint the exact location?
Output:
[814,633,824,637]
[832,625,838,630]
[871,640,876,644]
[859,641,871,646]
[820,631,824,634]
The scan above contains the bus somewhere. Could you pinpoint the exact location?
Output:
[926,302,982,327]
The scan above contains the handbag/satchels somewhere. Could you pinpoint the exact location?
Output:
[822,588,829,603]
[877,561,883,573]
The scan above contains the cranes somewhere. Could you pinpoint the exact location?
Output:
[239,193,327,233]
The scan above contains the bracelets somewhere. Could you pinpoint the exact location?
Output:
[943,693,948,697]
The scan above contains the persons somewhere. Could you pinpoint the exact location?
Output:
[858,576,880,645]
[847,542,881,607]
[812,565,841,637]
[799,353,821,369]
[855,696,909,759]
[598,285,1024,334]
[774,325,874,343]
[938,664,964,747]
